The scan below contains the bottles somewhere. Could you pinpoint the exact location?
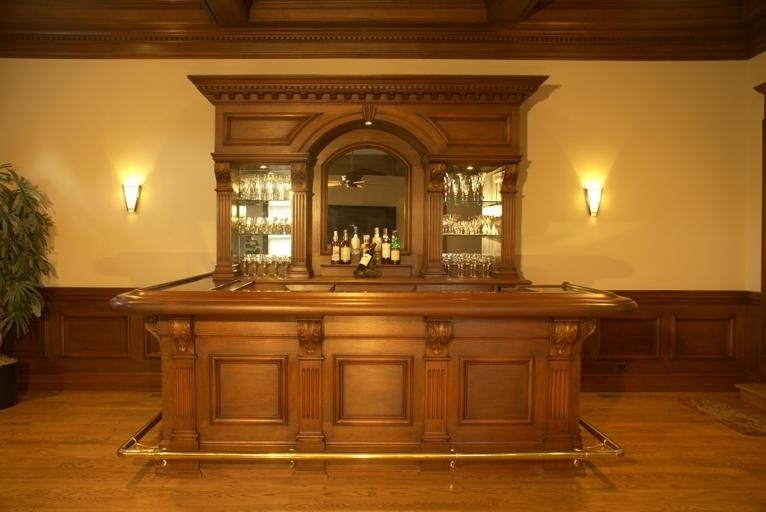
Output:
[330,225,402,265]
[357,242,377,269]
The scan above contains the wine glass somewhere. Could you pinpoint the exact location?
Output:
[442,213,502,236]
[441,171,485,202]
[440,251,495,272]
[240,252,291,280]
[235,216,289,235]
[237,174,291,201]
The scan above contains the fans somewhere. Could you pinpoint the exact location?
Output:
[326,148,375,195]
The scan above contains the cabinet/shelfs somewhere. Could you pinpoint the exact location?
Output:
[228,162,292,264]
[443,164,504,271]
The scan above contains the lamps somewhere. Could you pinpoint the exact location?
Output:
[120,182,143,216]
[583,186,606,217]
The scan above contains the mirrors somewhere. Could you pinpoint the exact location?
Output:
[318,138,415,256]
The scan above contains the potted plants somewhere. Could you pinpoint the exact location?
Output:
[1,162,60,410]
